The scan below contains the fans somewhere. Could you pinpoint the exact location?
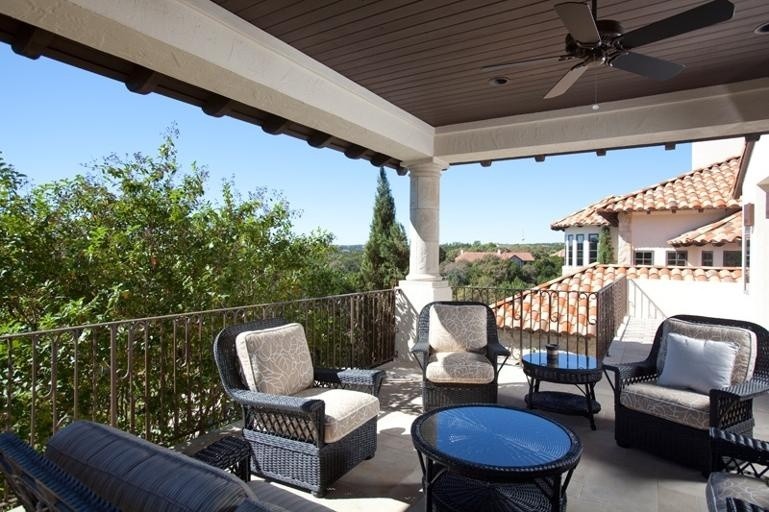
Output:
[469,0,736,113]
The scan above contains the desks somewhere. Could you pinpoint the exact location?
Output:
[409,404,585,512]
[521,352,603,432]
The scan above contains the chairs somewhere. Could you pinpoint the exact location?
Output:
[601,314,769,477]
[212,316,386,498]
[1,429,337,512]
[408,301,511,416]
[703,424,769,512]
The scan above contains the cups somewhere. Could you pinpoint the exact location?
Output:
[545,344,560,368]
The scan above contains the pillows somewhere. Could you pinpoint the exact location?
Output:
[653,331,740,397]
